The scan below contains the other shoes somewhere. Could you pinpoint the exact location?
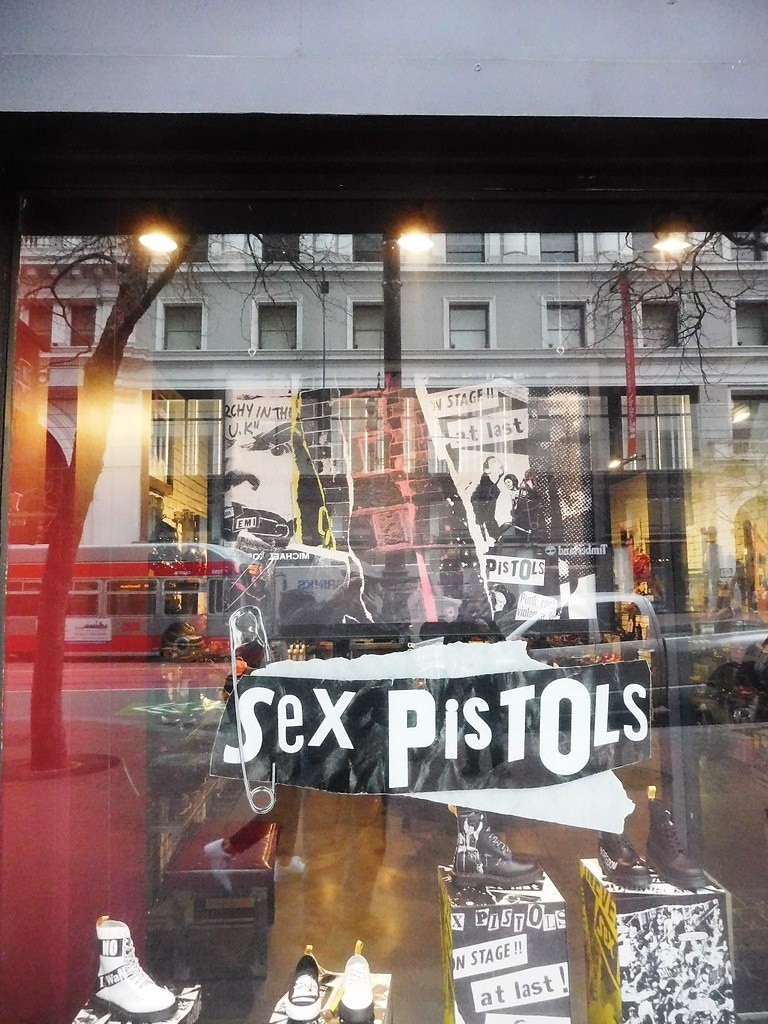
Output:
[286,944,322,1024]
[203,838,236,891]
[335,939,375,1024]
[273,855,306,884]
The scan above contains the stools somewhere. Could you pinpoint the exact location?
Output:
[158,812,278,983]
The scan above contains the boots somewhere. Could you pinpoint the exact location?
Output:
[447,804,544,887]
[644,786,707,889]
[87,915,179,1024]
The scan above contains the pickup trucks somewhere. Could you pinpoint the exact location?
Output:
[498,591,768,723]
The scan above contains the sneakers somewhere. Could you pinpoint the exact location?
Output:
[597,832,653,889]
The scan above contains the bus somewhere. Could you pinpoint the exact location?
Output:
[6,543,271,665]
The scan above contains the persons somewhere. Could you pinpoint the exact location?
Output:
[202,562,511,947]
[142,623,219,792]
[472,456,547,547]
